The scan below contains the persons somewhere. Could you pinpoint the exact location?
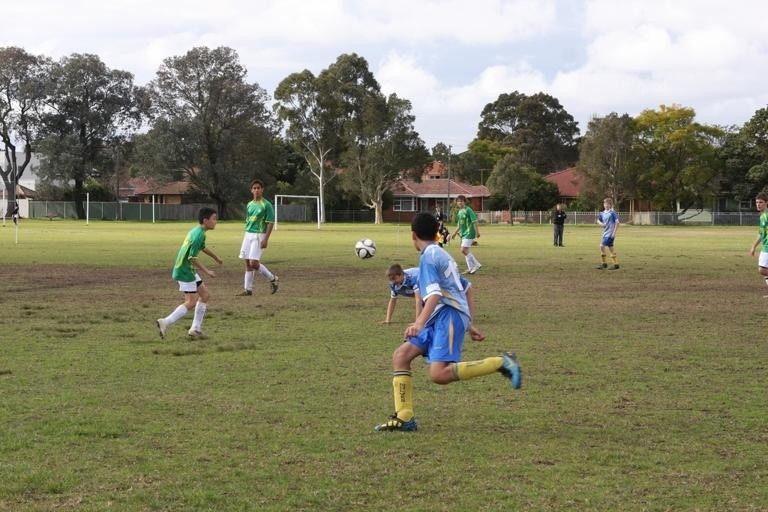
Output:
[11,201,19,226]
[451,195,482,275]
[380,263,423,324]
[233,178,279,296]
[749,191,768,299]
[594,197,620,270]
[432,202,443,249]
[156,206,224,340]
[551,203,567,247]
[372,212,524,430]
[442,227,451,246]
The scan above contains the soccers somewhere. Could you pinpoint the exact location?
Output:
[354,238,376,260]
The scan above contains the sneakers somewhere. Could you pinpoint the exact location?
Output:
[461,269,473,275]
[184,330,212,341]
[470,263,482,273]
[268,274,279,294]
[374,413,417,432]
[497,351,521,390]
[554,243,565,248]
[153,318,167,339]
[608,264,620,270]
[595,263,607,270]
[235,290,252,296]
